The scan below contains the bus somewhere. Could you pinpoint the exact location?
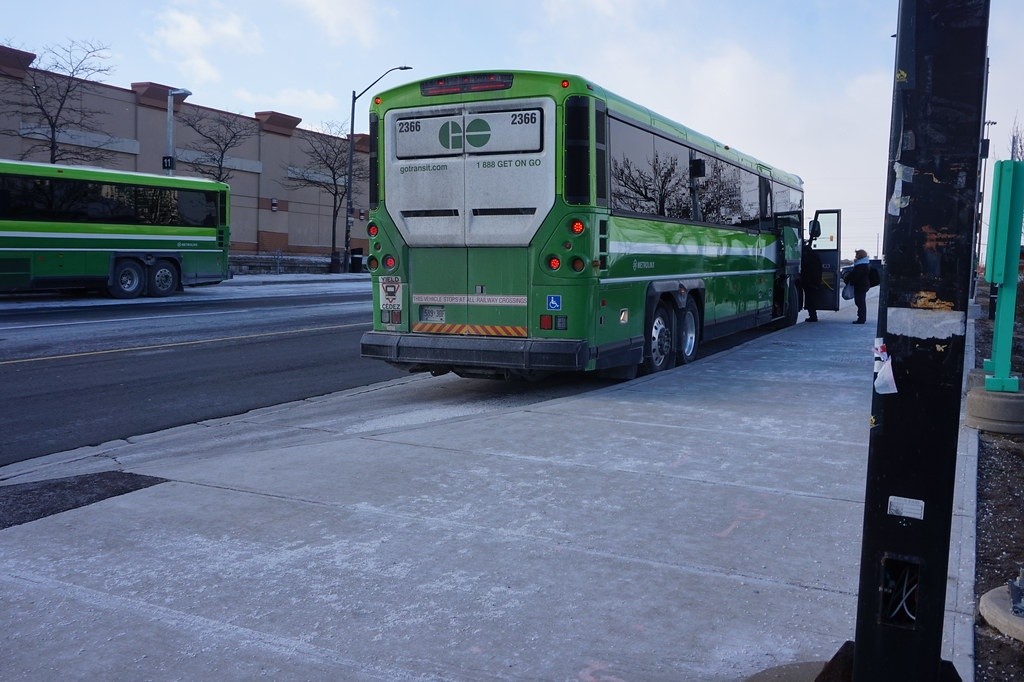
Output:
[0,157,236,298]
[357,66,843,381]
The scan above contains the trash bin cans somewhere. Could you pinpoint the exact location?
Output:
[350,247,363,273]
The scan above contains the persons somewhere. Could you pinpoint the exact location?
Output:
[802,245,823,322]
[844,250,871,324]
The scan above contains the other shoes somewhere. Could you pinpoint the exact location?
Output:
[805,317,818,322]
[853,320,865,324]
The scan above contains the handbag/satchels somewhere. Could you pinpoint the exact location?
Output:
[869,267,880,287]
[842,282,855,300]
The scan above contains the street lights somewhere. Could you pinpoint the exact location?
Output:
[975,120,997,272]
[342,64,414,274]
[166,88,193,176]
